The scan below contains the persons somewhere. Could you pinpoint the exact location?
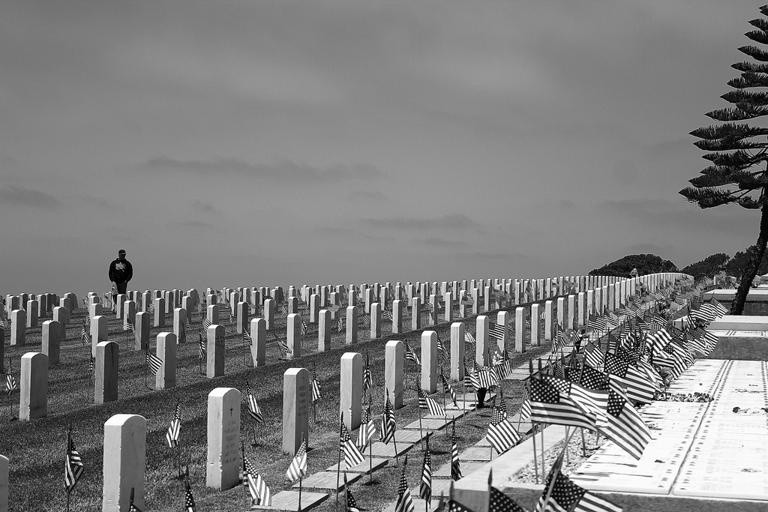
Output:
[109,249,133,294]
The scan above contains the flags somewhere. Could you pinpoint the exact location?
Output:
[2,294,731,512]
